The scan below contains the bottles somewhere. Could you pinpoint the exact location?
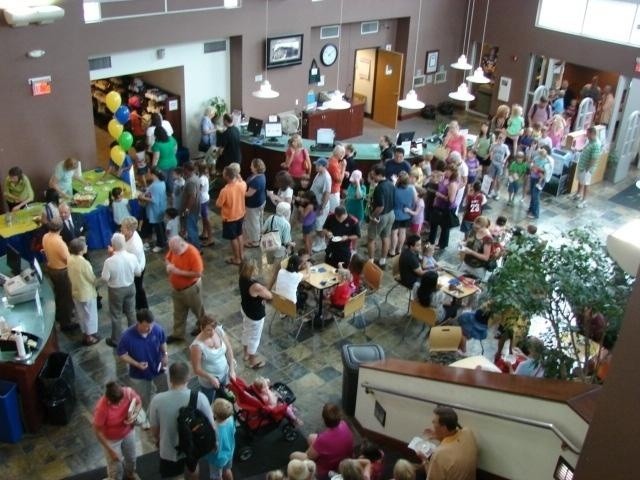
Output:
[313,158,327,167]
[349,170,362,182]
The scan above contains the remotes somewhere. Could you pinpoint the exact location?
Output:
[257,141,260,143]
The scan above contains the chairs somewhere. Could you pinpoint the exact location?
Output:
[191,328,201,336]
[166,335,184,344]
[106,338,117,347]
[87,335,100,345]
[142,419,150,429]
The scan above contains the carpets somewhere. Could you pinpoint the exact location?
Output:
[68,220,73,232]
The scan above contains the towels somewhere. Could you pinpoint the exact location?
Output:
[0,381,24,444]
[37,350,77,427]
[342,343,386,415]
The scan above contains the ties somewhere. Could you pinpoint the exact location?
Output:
[5,244,23,276]
[396,131,415,145]
[316,128,336,146]
[265,122,284,141]
[247,117,263,136]
[265,32,305,69]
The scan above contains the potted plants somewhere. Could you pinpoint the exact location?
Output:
[424,50,440,74]
[265,33,304,70]
[318,25,340,39]
[359,20,378,35]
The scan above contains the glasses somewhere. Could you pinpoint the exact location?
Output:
[448,1,476,102]
[321,0,352,111]
[465,1,492,85]
[450,0,472,70]
[396,0,427,110]
[251,1,281,99]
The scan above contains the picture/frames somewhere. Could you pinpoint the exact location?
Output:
[426,264,479,316]
[53,165,142,249]
[298,263,343,319]
[0,201,52,267]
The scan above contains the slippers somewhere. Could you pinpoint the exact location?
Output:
[174,391,216,461]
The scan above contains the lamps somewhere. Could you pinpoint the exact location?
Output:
[385,252,416,309]
[270,294,317,341]
[352,261,383,328]
[323,288,369,338]
[406,301,439,342]
[426,324,465,357]
[466,300,602,365]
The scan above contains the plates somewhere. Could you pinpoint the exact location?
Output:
[126,81,180,145]
[91,75,125,133]
[541,124,609,197]
[336,103,363,140]
[302,109,338,141]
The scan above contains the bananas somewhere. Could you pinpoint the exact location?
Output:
[311,144,336,152]
[262,142,284,147]
[239,135,257,143]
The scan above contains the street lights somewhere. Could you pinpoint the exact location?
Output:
[260,230,281,252]
[199,139,211,153]
[135,405,146,424]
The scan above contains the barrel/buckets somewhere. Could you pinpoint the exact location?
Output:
[250,361,265,369]
[226,259,243,265]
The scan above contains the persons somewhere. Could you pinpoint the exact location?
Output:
[130,95,181,174]
[0,157,218,344]
[201,104,216,175]
[239,239,548,379]
[218,76,617,265]
[94,311,478,479]
[106,139,131,176]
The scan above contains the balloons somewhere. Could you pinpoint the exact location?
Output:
[319,44,338,66]
[106,91,133,178]
